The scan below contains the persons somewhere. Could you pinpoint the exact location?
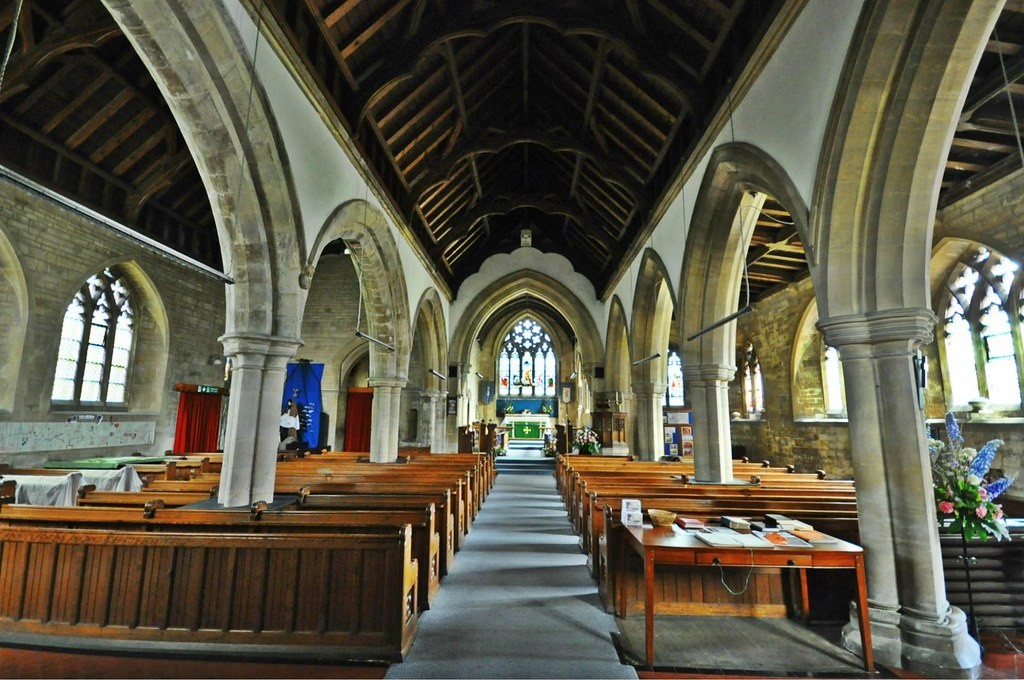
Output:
[284,398,300,439]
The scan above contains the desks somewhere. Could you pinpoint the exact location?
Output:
[620,522,873,672]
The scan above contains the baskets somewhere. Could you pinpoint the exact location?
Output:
[648,508,677,526]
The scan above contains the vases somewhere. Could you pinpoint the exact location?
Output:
[579,445,592,456]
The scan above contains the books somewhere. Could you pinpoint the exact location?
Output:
[720,516,751,530]
[694,533,774,547]
[788,529,838,544]
[676,518,704,529]
[751,514,813,531]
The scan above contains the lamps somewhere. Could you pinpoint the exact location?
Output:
[0,164,235,285]
[476,372,483,379]
[632,353,660,365]
[429,369,444,380]
[355,331,395,352]
[687,304,753,342]
[569,371,576,379]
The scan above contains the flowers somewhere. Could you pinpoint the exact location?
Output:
[542,437,558,457]
[502,404,515,414]
[541,404,553,414]
[567,425,602,455]
[494,434,507,456]
[926,411,1018,542]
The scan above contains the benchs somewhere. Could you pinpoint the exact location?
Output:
[0,449,497,663]
[554,450,862,617]
